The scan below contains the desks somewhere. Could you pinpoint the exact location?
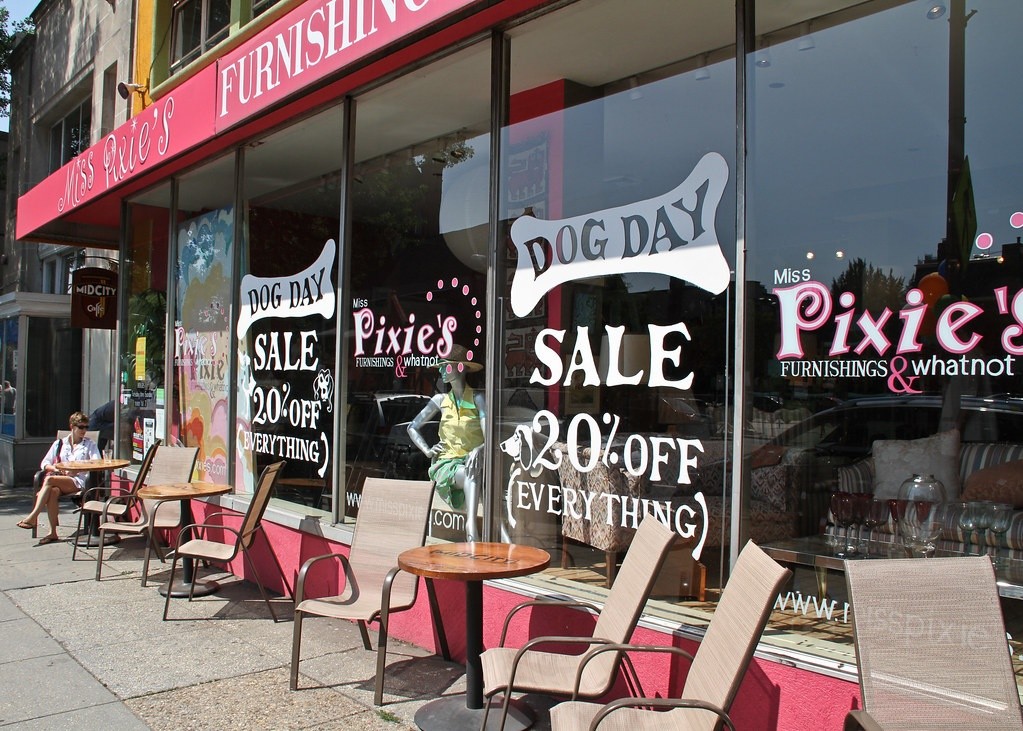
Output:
[56,459,131,546]
[397,542,552,731]
[136,483,233,597]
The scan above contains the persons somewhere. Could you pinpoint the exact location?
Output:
[16,412,102,544]
[87,400,115,458]
[406,344,513,543]
[0,381,16,414]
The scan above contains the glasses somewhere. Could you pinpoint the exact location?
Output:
[74,424,90,430]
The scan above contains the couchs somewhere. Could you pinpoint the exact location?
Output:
[558,432,810,587]
[825,438,1023,561]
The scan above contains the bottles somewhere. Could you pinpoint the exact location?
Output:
[897,474,947,551]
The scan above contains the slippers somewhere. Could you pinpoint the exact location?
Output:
[40,536,59,544]
[17,520,37,529]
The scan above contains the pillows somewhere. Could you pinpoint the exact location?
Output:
[962,459,1023,507]
[871,428,961,501]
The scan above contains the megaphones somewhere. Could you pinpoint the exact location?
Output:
[117,82,139,99]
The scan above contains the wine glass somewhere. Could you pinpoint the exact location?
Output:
[830,491,1013,570]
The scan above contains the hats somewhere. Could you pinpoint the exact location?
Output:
[427,343,483,372]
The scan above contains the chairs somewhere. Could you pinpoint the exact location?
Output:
[32,430,101,539]
[290,477,451,706]
[94,445,209,587]
[72,440,161,560]
[843,555,1023,731]
[163,460,295,622]
[549,539,793,731]
[478,512,680,731]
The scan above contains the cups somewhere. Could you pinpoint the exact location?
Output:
[102,450,113,464]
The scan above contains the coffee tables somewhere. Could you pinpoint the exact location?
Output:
[759,532,1023,600]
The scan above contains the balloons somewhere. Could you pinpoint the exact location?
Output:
[900,273,968,334]
